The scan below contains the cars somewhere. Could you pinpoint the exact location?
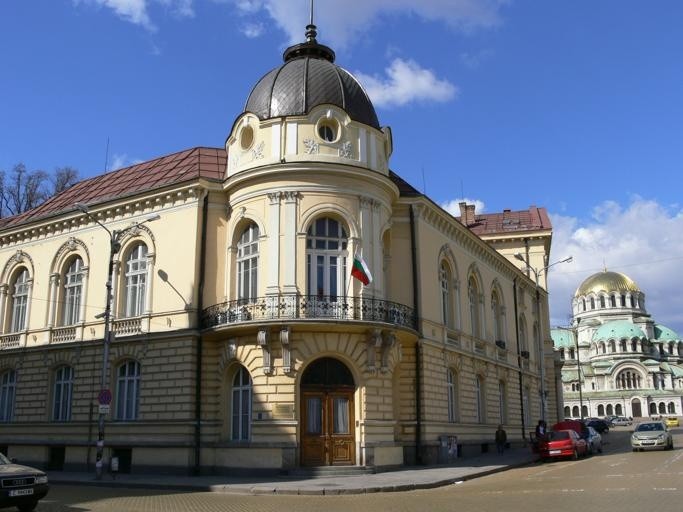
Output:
[0,449,49,511]
[527,412,679,460]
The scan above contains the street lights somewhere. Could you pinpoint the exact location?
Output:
[71,200,165,481]
[555,316,602,422]
[512,247,575,428]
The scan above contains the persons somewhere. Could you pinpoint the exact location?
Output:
[535,420,544,439]
[495,424,505,455]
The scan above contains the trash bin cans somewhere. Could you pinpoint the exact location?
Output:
[439,435,457,464]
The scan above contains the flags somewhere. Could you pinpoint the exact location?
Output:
[350,252,372,285]
